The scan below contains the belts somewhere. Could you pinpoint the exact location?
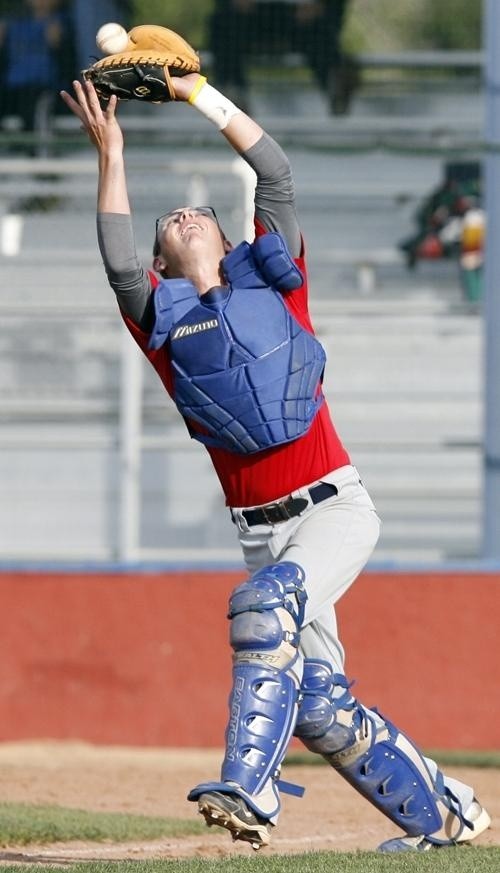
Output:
[232,483,337,529]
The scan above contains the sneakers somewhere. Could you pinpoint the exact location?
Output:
[376,798,490,855]
[199,787,272,850]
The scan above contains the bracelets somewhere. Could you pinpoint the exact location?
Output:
[187,76,208,103]
[191,81,241,130]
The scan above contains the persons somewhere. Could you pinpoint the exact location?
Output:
[402,154,485,306]
[2,0,71,133]
[206,0,368,118]
[59,23,495,852]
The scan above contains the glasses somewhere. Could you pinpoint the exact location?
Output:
[154,206,221,246]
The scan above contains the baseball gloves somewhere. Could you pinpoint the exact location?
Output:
[81,24,201,104]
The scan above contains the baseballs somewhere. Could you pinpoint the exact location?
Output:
[96,22,129,55]
